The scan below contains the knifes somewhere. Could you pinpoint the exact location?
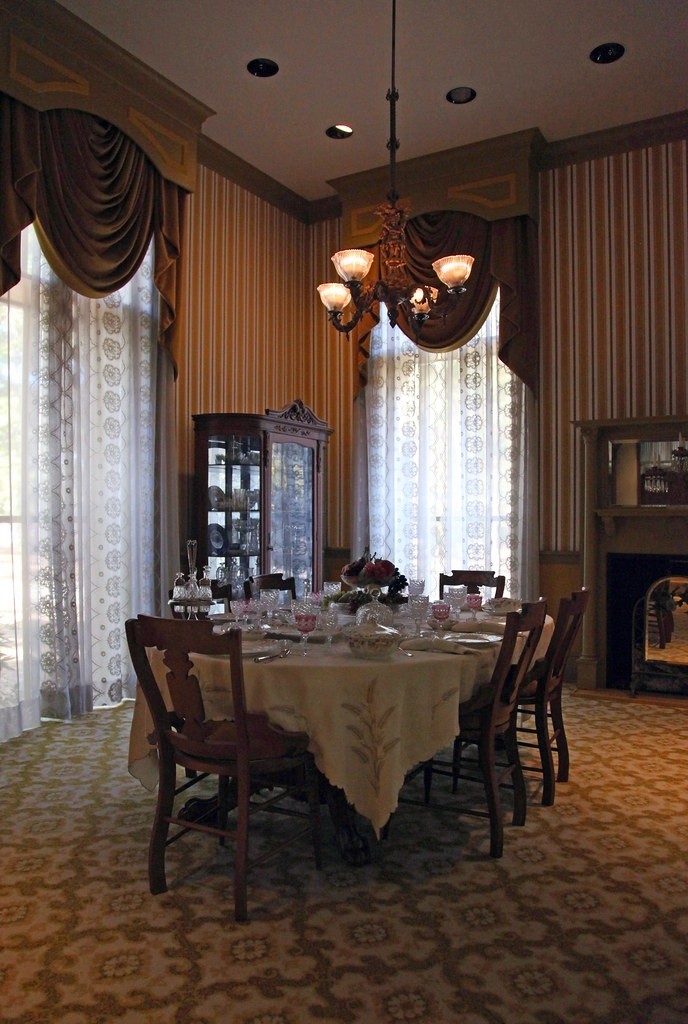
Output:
[254,647,290,663]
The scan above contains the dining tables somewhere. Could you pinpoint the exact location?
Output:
[128,612,554,867]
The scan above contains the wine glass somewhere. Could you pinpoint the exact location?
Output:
[228,578,483,657]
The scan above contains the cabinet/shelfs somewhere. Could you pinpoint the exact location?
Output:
[571,417,688,692]
[192,401,334,600]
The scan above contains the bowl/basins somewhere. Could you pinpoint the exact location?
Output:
[339,625,407,660]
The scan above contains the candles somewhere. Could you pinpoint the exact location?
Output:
[678,431,682,445]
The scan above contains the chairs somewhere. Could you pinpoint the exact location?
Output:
[170,573,296,614]
[451,586,590,806]
[126,618,321,922]
[378,596,548,856]
[439,570,506,601]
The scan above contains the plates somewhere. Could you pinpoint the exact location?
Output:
[209,486,225,509]
[207,523,226,555]
[443,633,503,648]
[481,608,522,616]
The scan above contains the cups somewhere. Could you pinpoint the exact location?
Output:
[215,489,260,585]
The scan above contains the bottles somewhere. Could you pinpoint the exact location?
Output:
[172,572,213,613]
[203,566,212,587]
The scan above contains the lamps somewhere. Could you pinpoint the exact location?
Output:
[316,1,478,332]
